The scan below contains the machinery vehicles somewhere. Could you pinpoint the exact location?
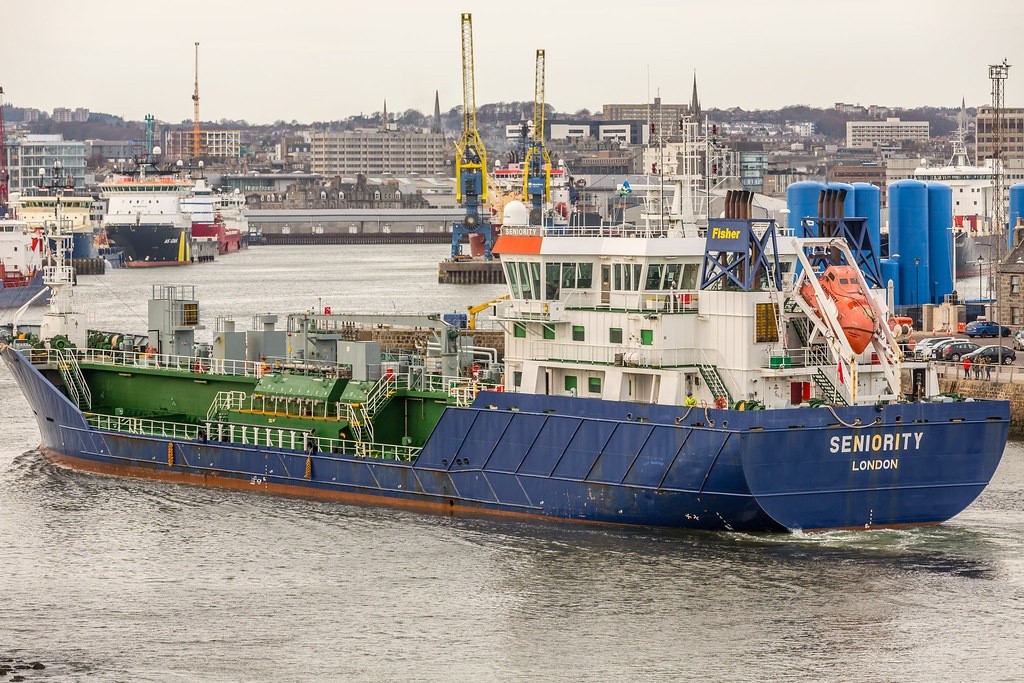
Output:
[142,114,155,162]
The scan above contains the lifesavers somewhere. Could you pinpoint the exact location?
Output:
[715,398,727,410]
[194,361,206,373]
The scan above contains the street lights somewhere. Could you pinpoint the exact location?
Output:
[912,255,921,332]
[35,160,77,222]
[978,254,986,302]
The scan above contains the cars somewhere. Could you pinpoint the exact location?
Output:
[963,321,1011,338]
[960,344,1017,365]
[1012,329,1024,351]
[941,341,981,363]
[912,336,956,356]
[920,339,969,361]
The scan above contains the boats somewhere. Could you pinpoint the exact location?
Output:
[97,163,251,267]
[0,217,42,289]
[0,192,1017,553]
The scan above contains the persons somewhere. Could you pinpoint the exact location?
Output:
[685,392,697,406]
[963,356,991,381]
[716,396,727,409]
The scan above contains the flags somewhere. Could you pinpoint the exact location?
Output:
[838,362,844,384]
[32,239,38,251]
